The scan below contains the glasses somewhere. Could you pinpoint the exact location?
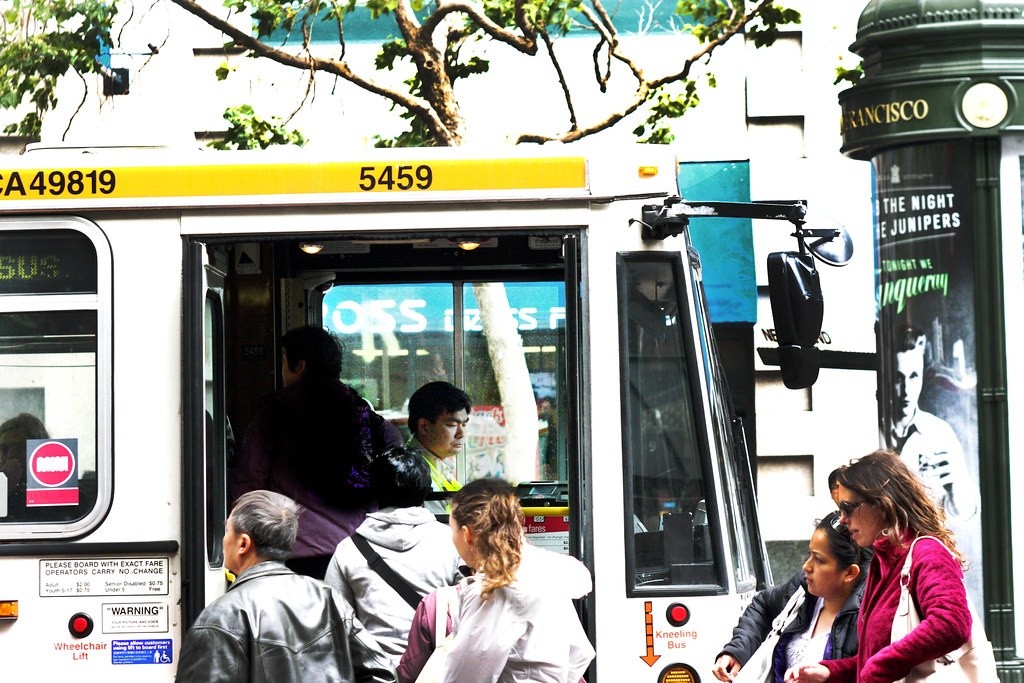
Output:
[839,500,874,517]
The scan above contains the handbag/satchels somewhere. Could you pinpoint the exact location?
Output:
[732,585,807,683]
[891,536,1000,683]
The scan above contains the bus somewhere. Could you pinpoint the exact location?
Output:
[0,144,854,683]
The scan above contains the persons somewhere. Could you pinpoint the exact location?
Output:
[0,413,65,521]
[176,491,399,683]
[784,450,974,683]
[396,479,595,683]
[229,324,403,580]
[878,326,978,529]
[828,467,845,508]
[712,510,873,683]
[405,380,472,524]
[324,446,472,683]
